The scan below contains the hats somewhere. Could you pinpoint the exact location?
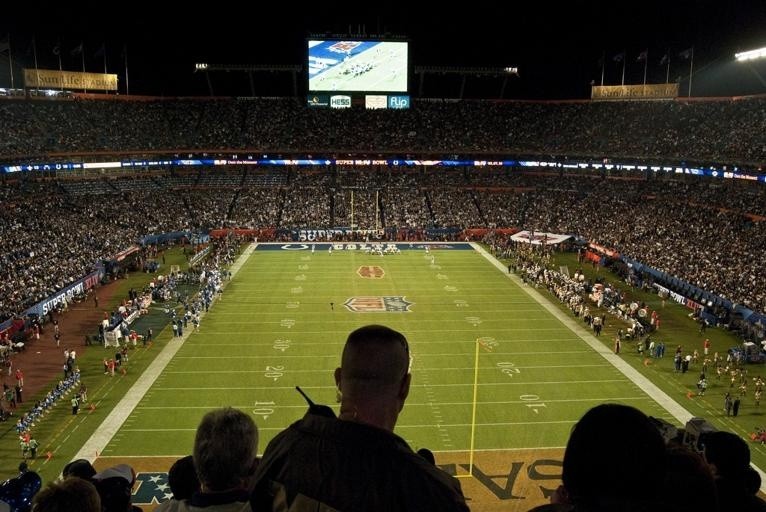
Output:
[63,459,98,485]
[0,471,41,512]
[93,464,136,488]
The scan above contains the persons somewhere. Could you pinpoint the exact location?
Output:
[1,96,766,174]
[0,324,766,512]
[488,163,766,444]
[1,167,244,460]
[234,164,534,242]
